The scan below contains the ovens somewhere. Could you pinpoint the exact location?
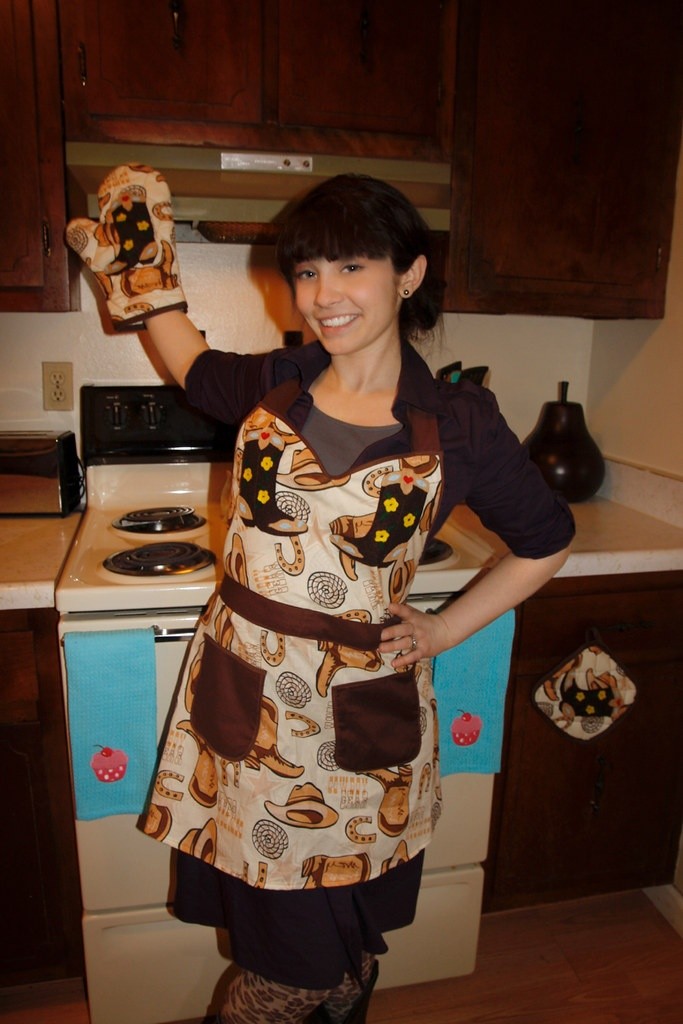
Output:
[59,611,520,1024]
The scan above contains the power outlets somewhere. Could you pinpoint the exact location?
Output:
[42,361,73,411]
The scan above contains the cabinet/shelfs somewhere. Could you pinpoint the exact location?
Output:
[480,570,683,915]
[441,0,683,320]
[56,0,457,165]
[0,0,83,314]
[0,607,88,990]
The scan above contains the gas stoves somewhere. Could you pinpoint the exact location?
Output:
[56,383,527,608]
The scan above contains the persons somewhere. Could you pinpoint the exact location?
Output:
[64,161,575,1024]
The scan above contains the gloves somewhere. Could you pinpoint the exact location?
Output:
[67,162,188,330]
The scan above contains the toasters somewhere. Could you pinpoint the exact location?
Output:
[0,428,82,517]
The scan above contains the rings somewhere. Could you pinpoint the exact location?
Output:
[410,635,417,649]
